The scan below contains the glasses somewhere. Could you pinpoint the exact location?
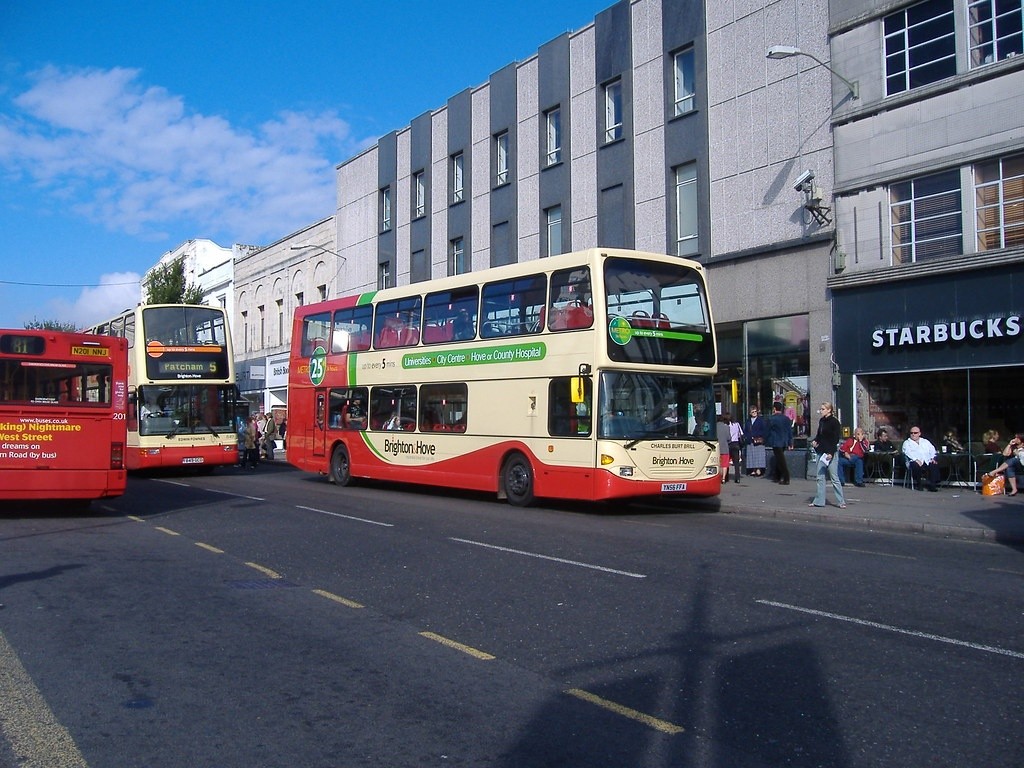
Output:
[910,432,919,434]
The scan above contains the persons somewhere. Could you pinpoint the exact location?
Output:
[808,402,847,509]
[692,412,709,436]
[716,402,796,486]
[140,394,166,420]
[872,429,905,483]
[774,394,809,439]
[988,432,1024,496]
[238,411,287,470]
[344,399,369,429]
[600,399,624,419]
[983,429,1004,455]
[902,425,939,492]
[838,427,870,487]
[942,426,965,453]
[454,308,491,340]
[382,415,404,430]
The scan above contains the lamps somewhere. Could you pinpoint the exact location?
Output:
[767,45,859,102]
[291,244,346,260]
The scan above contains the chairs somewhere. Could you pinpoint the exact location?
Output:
[404,423,416,431]
[445,319,454,342]
[484,327,503,337]
[204,340,218,345]
[432,423,451,432]
[348,332,360,352]
[651,313,670,329]
[961,441,1009,481]
[400,324,420,347]
[378,326,398,348]
[453,424,467,433]
[361,331,380,349]
[423,321,445,344]
[341,404,351,428]
[540,301,593,330]
[505,328,518,334]
[608,313,619,323]
[632,310,653,327]
[311,337,328,355]
[191,340,202,345]
[361,418,378,430]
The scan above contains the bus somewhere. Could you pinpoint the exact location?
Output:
[0,328,128,500]
[285,247,742,506]
[75,303,240,471]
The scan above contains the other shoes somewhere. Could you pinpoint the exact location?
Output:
[916,484,924,491]
[778,480,789,485]
[928,486,938,491]
[750,470,761,477]
[282,449,285,453]
[856,482,865,487]
[735,479,740,483]
[1009,491,1017,496]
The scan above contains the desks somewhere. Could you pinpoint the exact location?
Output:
[903,451,941,491]
[866,451,897,489]
[939,453,974,493]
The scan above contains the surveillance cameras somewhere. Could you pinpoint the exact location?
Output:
[792,169,815,191]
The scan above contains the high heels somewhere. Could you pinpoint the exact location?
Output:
[722,479,726,485]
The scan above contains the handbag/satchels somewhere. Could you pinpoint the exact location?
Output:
[745,443,766,469]
[982,473,1005,495]
[257,431,262,438]
[262,439,277,450]
[737,423,749,445]
